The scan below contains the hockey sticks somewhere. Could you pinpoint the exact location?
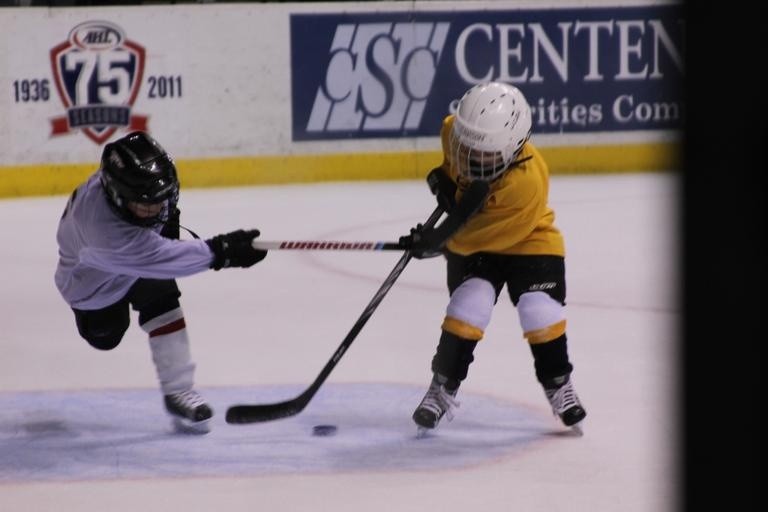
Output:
[224,205,445,425]
[252,181,489,252]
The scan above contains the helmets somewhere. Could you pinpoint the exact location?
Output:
[98,131,180,229]
[449,82,532,184]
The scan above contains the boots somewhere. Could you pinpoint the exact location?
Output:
[541,372,586,425]
[413,372,460,428]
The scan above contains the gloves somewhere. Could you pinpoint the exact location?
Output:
[427,168,458,214]
[400,224,445,259]
[205,229,267,270]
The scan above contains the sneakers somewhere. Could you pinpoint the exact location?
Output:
[164,391,212,422]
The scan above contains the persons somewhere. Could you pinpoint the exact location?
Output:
[397,80,589,431]
[52,128,268,423]
